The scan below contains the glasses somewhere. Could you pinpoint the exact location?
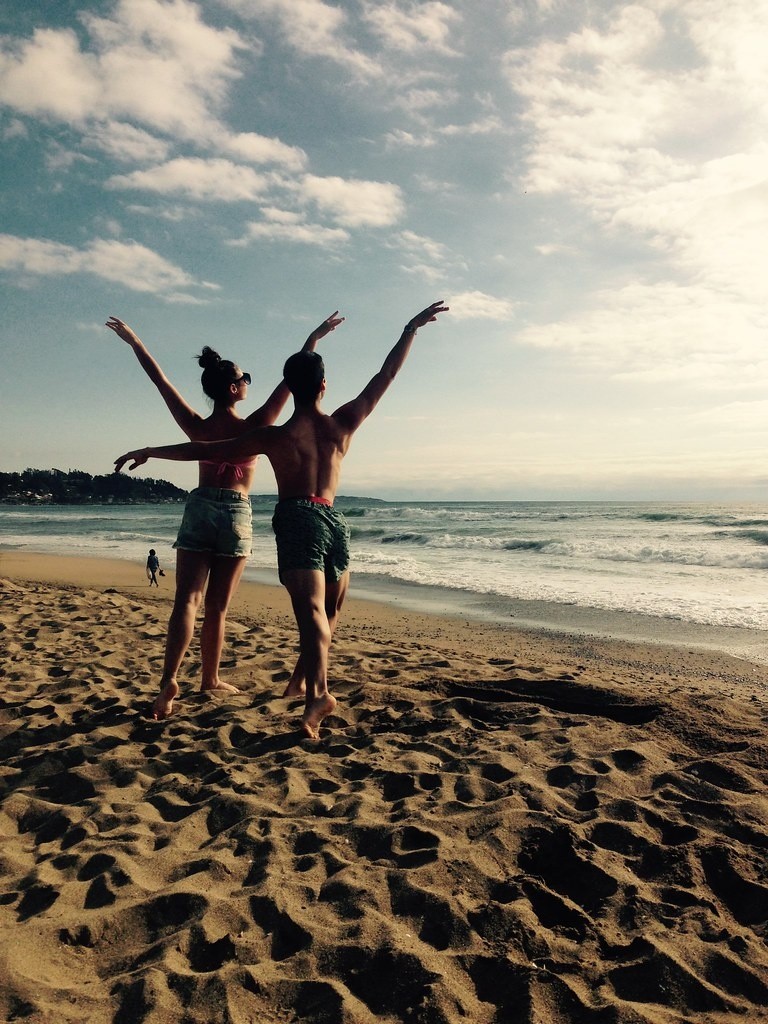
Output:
[236,371,252,386]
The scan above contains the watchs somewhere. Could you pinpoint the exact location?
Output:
[404,325,417,334]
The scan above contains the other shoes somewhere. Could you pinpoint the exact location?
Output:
[159,569,165,576]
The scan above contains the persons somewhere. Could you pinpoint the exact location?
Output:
[105,312,344,721]
[113,300,450,739]
[143,547,162,587]
